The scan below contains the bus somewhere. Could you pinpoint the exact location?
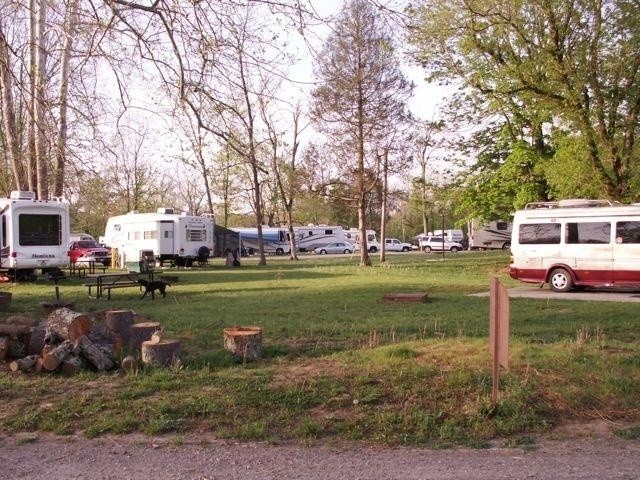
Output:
[507,197,640,293]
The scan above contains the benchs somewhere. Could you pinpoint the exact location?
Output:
[85,280,163,300]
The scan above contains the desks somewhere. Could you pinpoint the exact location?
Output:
[86,269,163,302]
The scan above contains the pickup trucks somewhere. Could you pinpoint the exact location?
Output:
[366,238,412,252]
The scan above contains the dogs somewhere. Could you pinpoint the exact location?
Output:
[137,278,172,301]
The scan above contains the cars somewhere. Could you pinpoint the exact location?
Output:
[71,240,112,266]
[314,240,354,255]
[418,235,463,253]
